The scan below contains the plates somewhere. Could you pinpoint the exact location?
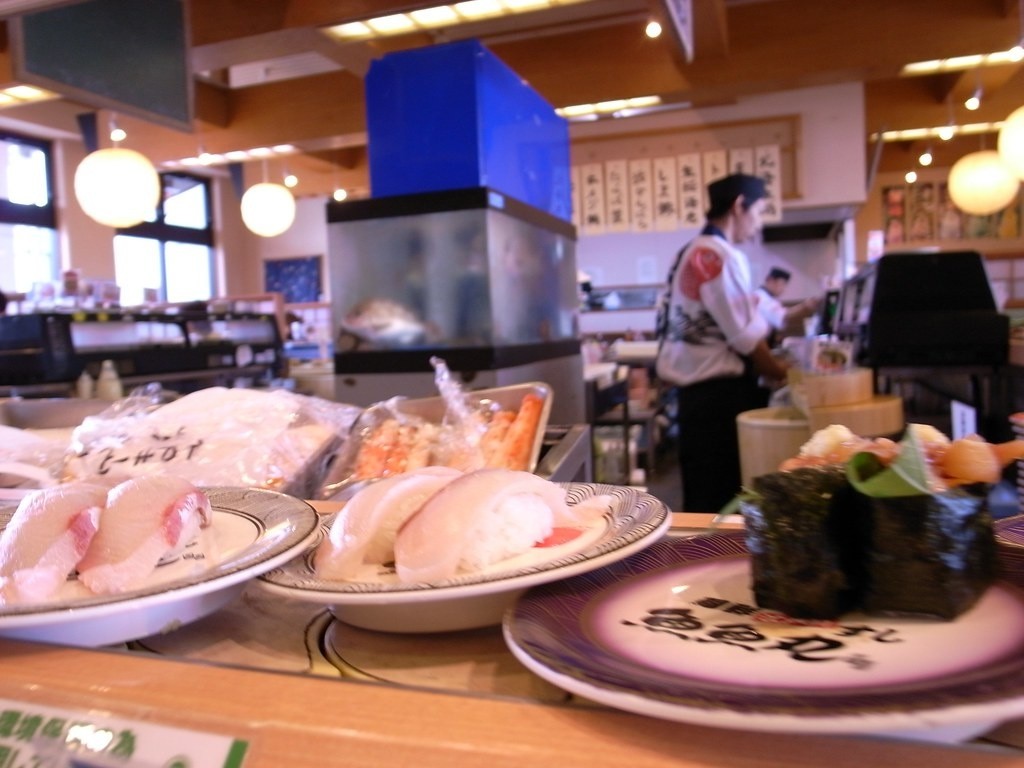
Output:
[499,529,1024,739]
[313,380,553,501]
[0,489,321,651]
[236,482,672,634]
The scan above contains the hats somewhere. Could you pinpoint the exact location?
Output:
[708,173,772,208]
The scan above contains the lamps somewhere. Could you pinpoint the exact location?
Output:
[74,113,161,227]
[966,89,984,111]
[107,120,127,142]
[939,120,959,142]
[643,16,662,39]
[281,168,299,189]
[330,183,348,201]
[919,146,933,168]
[947,128,1021,217]
[239,159,295,236]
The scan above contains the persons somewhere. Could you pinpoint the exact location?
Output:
[655,174,819,512]
[581,283,596,311]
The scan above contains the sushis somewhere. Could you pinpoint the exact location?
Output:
[0,472,211,607]
[714,422,996,621]
[310,465,611,584]
